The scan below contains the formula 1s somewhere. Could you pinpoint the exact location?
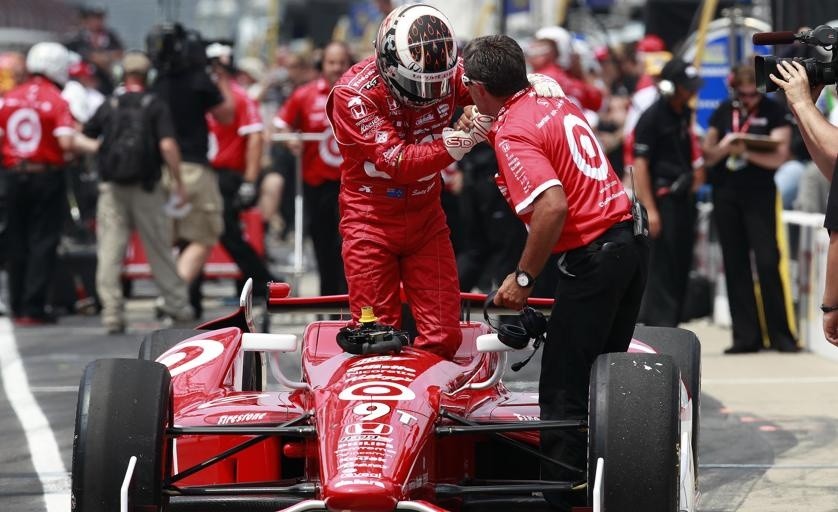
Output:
[59,274,716,512]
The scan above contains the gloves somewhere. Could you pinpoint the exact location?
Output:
[527,72,565,98]
[232,180,258,208]
[442,104,496,162]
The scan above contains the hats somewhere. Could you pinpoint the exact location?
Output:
[636,38,758,90]
[23,38,236,89]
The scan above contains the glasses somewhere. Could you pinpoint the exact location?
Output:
[461,73,485,88]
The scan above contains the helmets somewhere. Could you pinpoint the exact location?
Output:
[373,2,458,110]
[536,27,604,76]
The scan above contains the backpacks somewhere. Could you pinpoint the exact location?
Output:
[100,93,161,185]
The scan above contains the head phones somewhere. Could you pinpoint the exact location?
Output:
[112,51,159,88]
[315,40,358,73]
[483,292,548,350]
[656,63,699,98]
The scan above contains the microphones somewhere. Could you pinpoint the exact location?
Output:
[502,338,542,373]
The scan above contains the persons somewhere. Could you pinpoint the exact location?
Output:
[1,0,838,334]
[701,66,800,354]
[820,161,838,345]
[451,33,652,512]
[323,3,479,362]
